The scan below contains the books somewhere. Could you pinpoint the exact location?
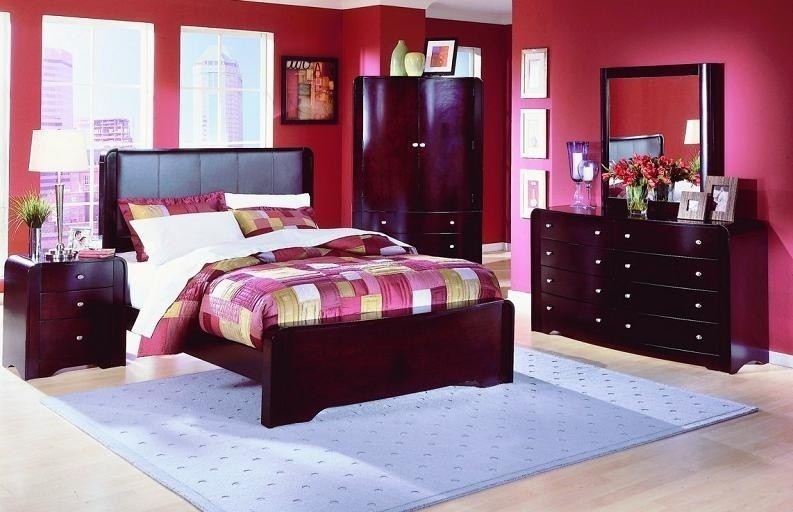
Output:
[78,248,116,259]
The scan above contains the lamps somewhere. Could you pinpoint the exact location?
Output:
[28,129,85,258]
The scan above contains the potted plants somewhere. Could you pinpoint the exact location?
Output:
[10,185,52,256]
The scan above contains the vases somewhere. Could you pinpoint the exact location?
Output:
[624,178,649,215]
[654,185,668,202]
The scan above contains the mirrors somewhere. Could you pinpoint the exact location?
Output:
[599,64,713,209]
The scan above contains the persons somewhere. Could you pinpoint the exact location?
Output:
[73,231,86,250]
[713,186,728,212]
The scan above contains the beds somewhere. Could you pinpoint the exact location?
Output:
[98,147,514,430]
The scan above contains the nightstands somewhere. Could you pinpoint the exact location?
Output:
[3,255,140,382]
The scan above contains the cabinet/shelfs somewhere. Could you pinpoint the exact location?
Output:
[362,78,466,259]
[535,212,721,358]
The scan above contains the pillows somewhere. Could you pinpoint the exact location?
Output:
[117,190,228,262]
[128,211,245,264]
[225,191,310,210]
[233,206,319,238]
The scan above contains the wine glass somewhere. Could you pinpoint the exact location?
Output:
[578,160,598,209]
[567,140,588,208]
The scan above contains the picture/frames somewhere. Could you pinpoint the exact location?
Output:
[68,227,92,253]
[519,108,547,159]
[705,175,738,223]
[520,47,548,99]
[424,38,457,75]
[281,54,337,125]
[519,169,546,219]
[677,191,707,222]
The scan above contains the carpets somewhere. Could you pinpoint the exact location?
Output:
[40,344,758,512]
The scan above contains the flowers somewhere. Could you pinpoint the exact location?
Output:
[600,155,672,189]
[657,155,700,186]
